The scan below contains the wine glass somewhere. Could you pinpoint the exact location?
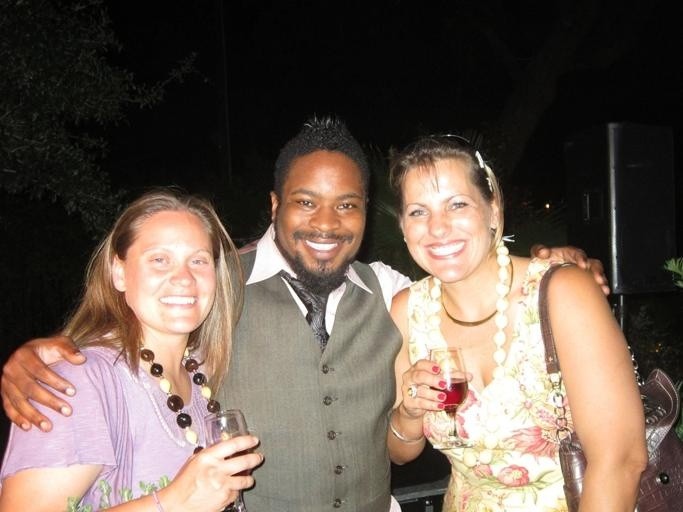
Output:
[202,406,256,511]
[425,348,478,456]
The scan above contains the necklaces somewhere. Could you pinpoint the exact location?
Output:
[135,343,223,456]
[430,240,511,382]
[439,236,517,328]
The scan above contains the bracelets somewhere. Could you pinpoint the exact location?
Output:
[150,490,165,512]
[389,408,425,444]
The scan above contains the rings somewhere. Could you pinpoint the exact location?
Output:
[407,383,418,399]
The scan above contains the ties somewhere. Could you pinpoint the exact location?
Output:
[274,267,330,353]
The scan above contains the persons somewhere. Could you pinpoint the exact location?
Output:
[0,184,268,512]
[385,132,651,512]
[1,118,612,512]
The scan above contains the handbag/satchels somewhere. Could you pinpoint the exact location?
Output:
[557,365,683,512]
